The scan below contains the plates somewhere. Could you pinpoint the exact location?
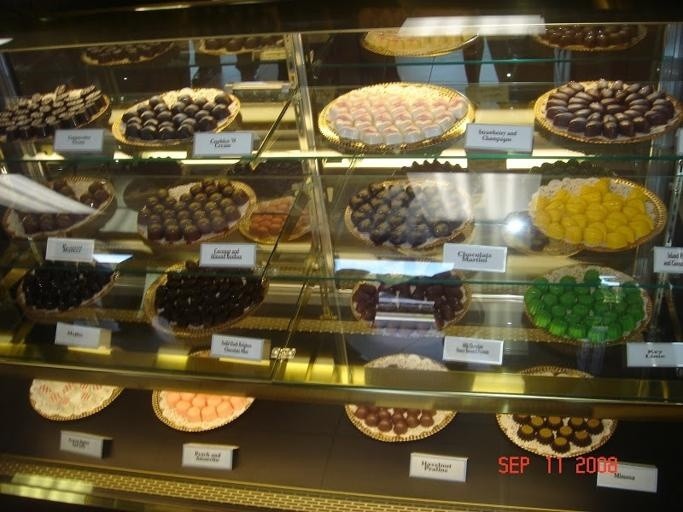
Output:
[496,365,618,459]
[143,256,269,342]
[344,353,458,443]
[111,88,240,151]
[317,81,475,155]
[528,176,667,253]
[137,177,253,247]
[534,79,683,144]
[238,195,311,242]
[360,24,649,59]
[28,376,124,423]
[149,389,256,432]
[13,262,120,317]
[350,259,473,334]
[13,89,110,141]
[2,176,118,242]
[345,177,475,249]
[523,263,653,347]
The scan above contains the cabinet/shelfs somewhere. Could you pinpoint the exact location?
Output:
[1,0,682,512]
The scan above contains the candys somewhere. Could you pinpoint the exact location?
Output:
[82,24,641,61]
[546,78,675,138]
[249,199,311,238]
[325,83,468,143]
[137,180,250,244]
[525,269,644,344]
[121,95,234,140]
[534,180,654,249]
[15,179,108,233]
[0,79,107,140]
[355,405,440,435]
[347,180,464,245]
[38,381,107,411]
[154,260,264,328]
[23,259,113,312]
[353,271,465,328]
[513,413,604,453]
[167,392,246,422]
